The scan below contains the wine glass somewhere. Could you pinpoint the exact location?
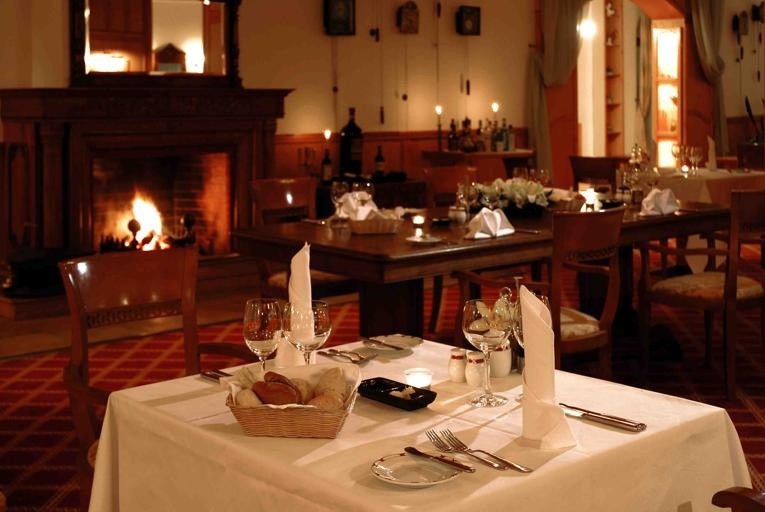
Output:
[283,301,334,366]
[616,166,660,205]
[631,141,703,177]
[242,298,283,379]
[459,166,552,221]
[513,294,552,403]
[461,298,512,408]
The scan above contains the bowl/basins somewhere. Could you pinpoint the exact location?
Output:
[332,179,375,227]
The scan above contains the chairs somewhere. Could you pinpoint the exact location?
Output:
[570,155,632,192]
[56,246,200,512]
[249,175,365,338]
[451,210,622,378]
[632,187,764,402]
[419,163,552,331]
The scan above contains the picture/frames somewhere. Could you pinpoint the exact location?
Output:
[322,0,358,36]
[456,5,480,35]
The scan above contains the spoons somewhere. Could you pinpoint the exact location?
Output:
[320,346,381,365]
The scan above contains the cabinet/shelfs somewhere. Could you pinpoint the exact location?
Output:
[0,85,298,316]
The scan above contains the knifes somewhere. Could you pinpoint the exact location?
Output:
[515,228,542,236]
[357,334,405,351]
[555,400,647,432]
[403,446,476,474]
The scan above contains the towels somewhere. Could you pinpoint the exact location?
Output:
[520,283,577,454]
[640,186,682,218]
[465,206,516,242]
[332,192,379,222]
[279,242,328,366]
[701,134,721,169]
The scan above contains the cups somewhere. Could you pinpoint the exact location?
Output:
[403,367,434,390]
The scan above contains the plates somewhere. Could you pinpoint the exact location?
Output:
[405,235,441,246]
[372,451,461,486]
[367,332,425,352]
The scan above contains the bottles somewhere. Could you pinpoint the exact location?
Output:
[321,148,333,184]
[464,351,487,388]
[445,116,516,153]
[489,339,511,378]
[448,206,466,222]
[340,107,365,179]
[372,143,386,177]
[448,347,467,382]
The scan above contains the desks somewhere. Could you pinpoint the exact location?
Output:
[422,149,535,196]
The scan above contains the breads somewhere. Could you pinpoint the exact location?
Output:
[236,366,352,414]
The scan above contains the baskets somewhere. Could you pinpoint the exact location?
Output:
[225,386,350,440]
[548,197,585,212]
[346,212,403,234]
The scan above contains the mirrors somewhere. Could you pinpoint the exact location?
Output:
[66,1,242,90]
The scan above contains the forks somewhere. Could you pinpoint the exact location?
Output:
[425,430,509,471]
[441,425,533,474]
